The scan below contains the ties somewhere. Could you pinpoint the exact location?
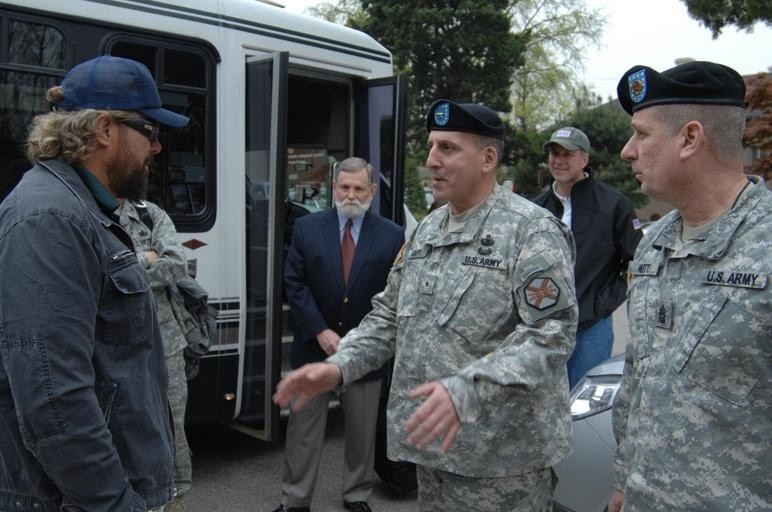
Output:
[341,218,355,289]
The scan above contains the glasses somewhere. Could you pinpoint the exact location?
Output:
[115,117,161,142]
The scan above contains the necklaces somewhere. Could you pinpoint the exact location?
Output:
[727,178,752,213]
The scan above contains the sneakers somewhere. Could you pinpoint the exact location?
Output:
[164,495,184,512]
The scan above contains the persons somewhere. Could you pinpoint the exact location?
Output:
[532,123,644,391]
[0,54,194,512]
[114,189,192,511]
[269,99,579,510]
[275,156,406,512]
[603,62,771,512]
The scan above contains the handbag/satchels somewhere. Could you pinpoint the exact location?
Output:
[164,276,218,356]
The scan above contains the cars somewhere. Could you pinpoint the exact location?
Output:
[550,355,623,511]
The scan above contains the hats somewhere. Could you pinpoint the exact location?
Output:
[52,54,190,127]
[617,62,749,116]
[426,99,505,140]
[543,126,591,152]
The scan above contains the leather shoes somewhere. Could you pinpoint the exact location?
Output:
[343,499,371,512]
[271,504,310,512]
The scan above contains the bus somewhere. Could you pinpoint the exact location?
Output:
[0,0,406,445]
